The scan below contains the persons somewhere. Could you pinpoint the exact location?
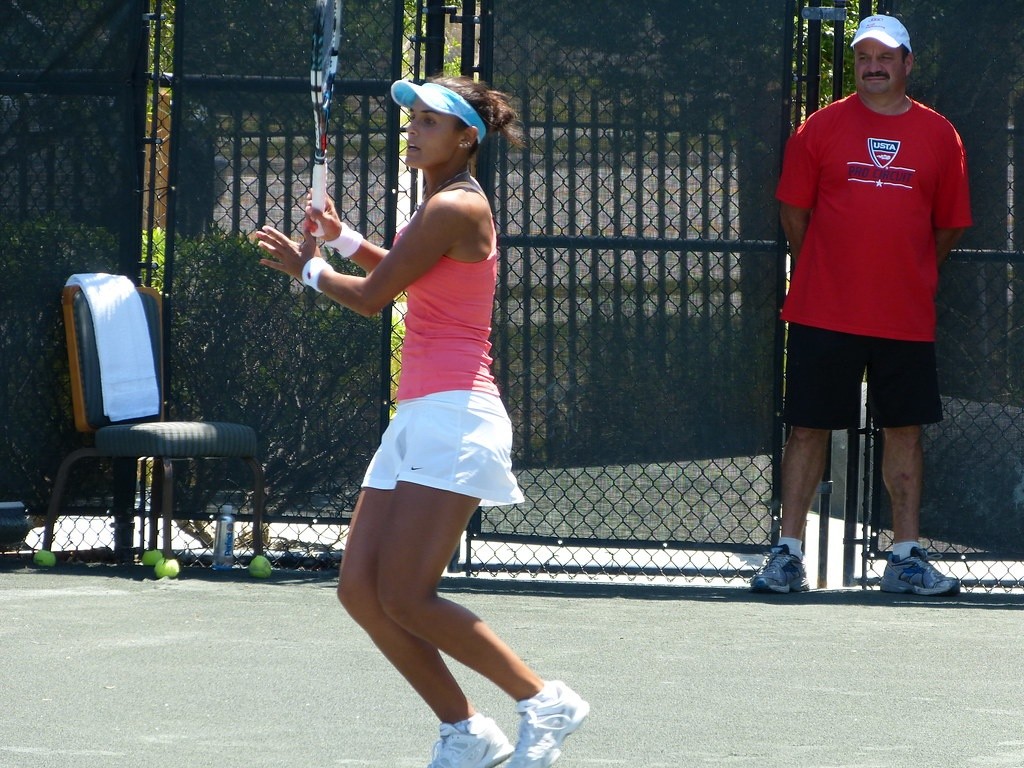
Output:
[750,14,973,597]
[255,75,592,768]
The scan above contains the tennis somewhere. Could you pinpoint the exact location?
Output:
[33,550,56,567]
[154,558,180,579]
[142,549,163,566]
[248,555,272,578]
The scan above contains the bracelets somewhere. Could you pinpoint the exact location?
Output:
[326,222,363,258]
[302,257,334,293]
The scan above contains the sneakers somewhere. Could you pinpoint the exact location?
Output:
[749,544,810,593]
[505,680,590,768]
[426,717,516,768]
[880,546,960,595]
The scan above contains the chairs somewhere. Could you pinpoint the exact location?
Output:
[35,281,273,582]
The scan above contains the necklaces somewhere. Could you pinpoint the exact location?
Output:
[415,170,469,212]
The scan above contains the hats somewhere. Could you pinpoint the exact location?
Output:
[390,79,487,145]
[850,14,912,53]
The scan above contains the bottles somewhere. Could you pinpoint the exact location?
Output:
[212,505,234,570]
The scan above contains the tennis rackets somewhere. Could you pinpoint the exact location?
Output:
[305,0,344,238]
